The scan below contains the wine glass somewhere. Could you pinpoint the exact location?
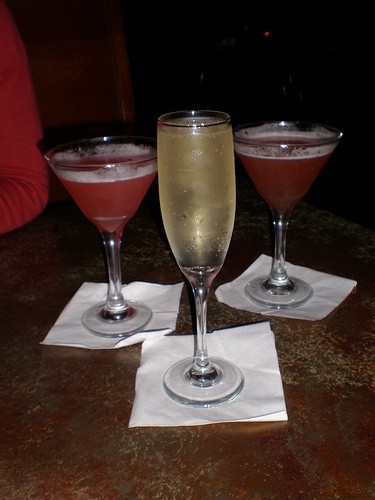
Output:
[46,135,159,337]
[155,109,246,408]
[229,124,342,309]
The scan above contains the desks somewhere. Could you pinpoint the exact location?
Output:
[0,192,375,499]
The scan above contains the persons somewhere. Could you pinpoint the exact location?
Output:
[0,0,51,234]
